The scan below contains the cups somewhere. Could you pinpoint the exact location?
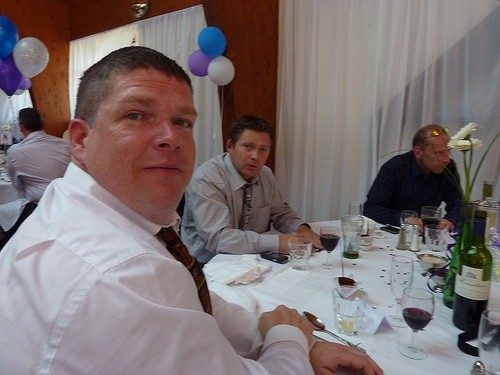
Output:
[401,210,419,245]
[425,224,448,254]
[288,237,313,270]
[342,216,364,259]
[421,206,442,245]
[417,250,452,296]
[477,310,500,375]
[358,220,375,251]
[332,287,368,336]
[348,203,364,216]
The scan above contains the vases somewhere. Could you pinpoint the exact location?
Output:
[444,204,473,309]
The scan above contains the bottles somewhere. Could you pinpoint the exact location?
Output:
[396,224,408,249]
[409,224,420,251]
[452,211,493,332]
[471,361,485,375]
[472,182,500,310]
[443,207,474,309]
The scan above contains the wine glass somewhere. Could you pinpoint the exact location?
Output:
[320,225,341,268]
[387,256,414,327]
[400,287,435,360]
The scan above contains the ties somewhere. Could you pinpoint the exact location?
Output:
[239,183,254,231]
[156,226,213,317]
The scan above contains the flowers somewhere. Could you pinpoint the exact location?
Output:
[374,122,500,213]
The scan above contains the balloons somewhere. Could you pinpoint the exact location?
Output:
[0,17,49,96]
[188,27,234,86]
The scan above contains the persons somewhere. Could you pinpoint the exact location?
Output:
[6,107,72,204]
[178,114,324,266]
[364,125,468,241]
[0,46,384,375]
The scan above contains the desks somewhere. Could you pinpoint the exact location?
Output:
[0,149,28,232]
[201,218,500,375]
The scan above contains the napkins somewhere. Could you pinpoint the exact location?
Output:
[204,262,260,285]
[353,215,386,240]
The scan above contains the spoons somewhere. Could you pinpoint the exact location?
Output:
[303,311,366,353]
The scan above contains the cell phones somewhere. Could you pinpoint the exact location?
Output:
[261,249,289,264]
[381,226,400,234]
